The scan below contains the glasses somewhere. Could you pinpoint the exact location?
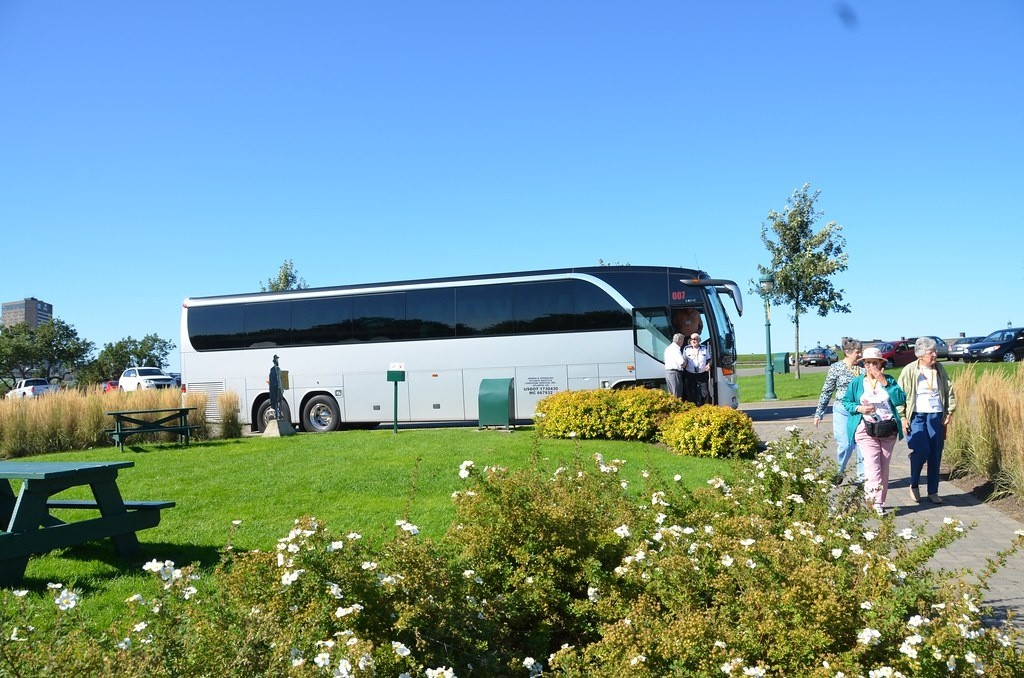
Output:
[865,361,877,365]
[691,339,698,341]
[925,348,939,356]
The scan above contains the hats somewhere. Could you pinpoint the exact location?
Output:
[856,348,890,365]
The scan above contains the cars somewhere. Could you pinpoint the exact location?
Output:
[962,327,1024,362]
[93,367,181,393]
[874,336,951,370]
[801,348,839,367]
[948,336,987,363]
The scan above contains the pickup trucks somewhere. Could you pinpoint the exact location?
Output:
[5,378,61,401]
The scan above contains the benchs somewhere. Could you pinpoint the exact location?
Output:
[104,425,201,442]
[15,496,176,533]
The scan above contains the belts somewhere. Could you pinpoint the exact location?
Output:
[667,369,680,372]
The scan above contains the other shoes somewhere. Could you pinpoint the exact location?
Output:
[874,506,888,518]
[836,472,843,484]
[928,493,943,504]
[854,481,865,490]
[910,485,920,502]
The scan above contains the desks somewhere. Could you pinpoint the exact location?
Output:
[104,407,198,451]
[0,461,143,589]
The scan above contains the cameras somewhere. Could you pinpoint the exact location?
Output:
[871,412,882,424]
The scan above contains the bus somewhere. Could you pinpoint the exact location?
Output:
[181,266,742,432]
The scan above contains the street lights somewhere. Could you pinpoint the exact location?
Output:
[759,267,779,401]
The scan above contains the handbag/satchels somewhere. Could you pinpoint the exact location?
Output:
[865,421,897,437]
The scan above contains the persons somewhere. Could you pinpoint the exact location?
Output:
[269,355,284,420]
[897,337,956,503]
[683,334,712,404]
[674,305,703,346]
[814,339,865,483]
[842,348,906,514]
[665,333,686,399]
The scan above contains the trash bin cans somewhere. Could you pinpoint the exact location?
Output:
[478,378,516,431]
[773,352,789,375]
